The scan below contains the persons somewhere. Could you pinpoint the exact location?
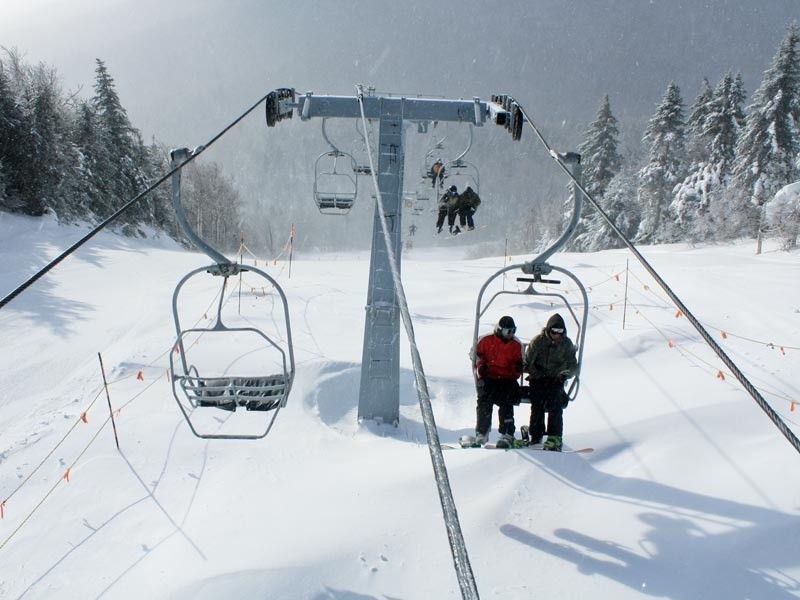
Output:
[524,313,578,448]
[431,158,445,189]
[470,316,525,448]
[436,185,460,228]
[456,186,481,231]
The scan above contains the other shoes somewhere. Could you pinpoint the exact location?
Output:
[449,224,454,231]
[437,224,443,231]
[544,437,562,450]
[496,431,514,449]
[460,224,465,227]
[467,226,475,230]
[529,432,547,445]
[476,429,491,445]
[431,184,436,188]
[440,185,444,188]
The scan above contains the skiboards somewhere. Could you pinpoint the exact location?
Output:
[431,224,489,241]
[437,443,594,454]
[428,186,447,191]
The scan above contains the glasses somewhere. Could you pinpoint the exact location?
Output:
[500,327,517,335]
[549,327,565,336]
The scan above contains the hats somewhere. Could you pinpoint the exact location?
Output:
[437,158,441,164]
[499,315,516,329]
[466,186,474,193]
[546,313,566,332]
[450,185,457,191]
